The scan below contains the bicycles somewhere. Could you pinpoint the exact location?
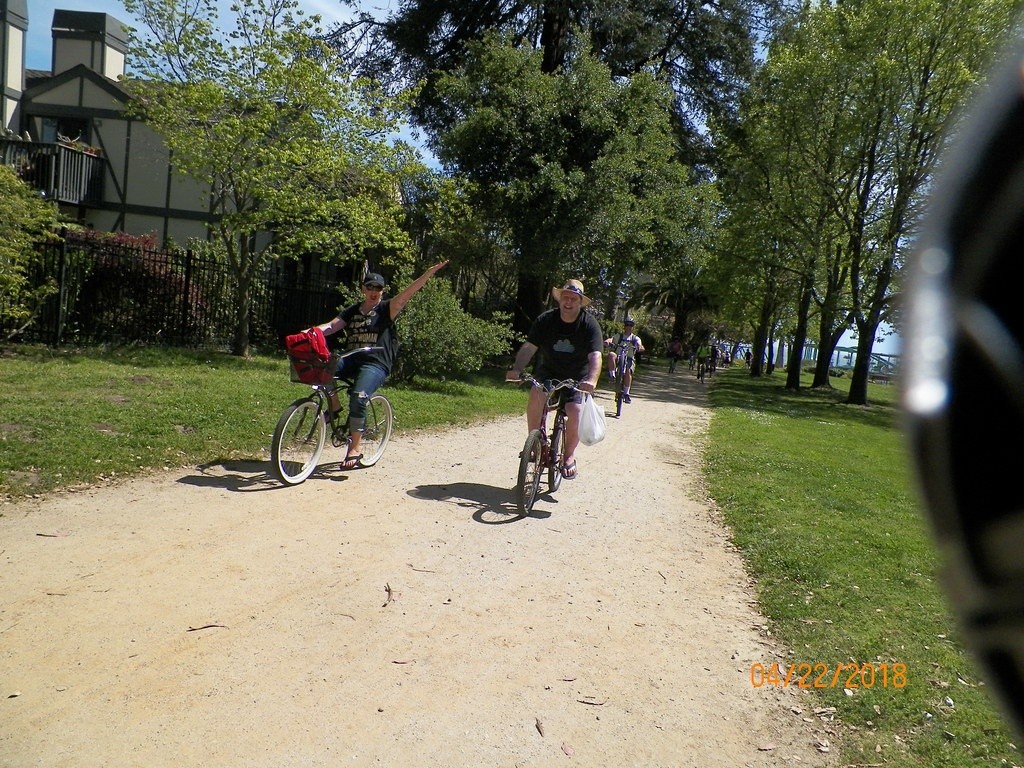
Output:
[667,354,750,383]
[603,342,636,417]
[505,372,591,517]
[272,347,392,486]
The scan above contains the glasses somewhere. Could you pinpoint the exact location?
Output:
[365,284,383,292]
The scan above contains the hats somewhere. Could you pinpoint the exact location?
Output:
[552,279,591,307]
[363,273,385,288]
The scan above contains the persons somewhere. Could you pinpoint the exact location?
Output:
[604,316,645,404]
[506,279,605,479]
[666,336,683,373]
[689,338,730,379]
[745,349,753,367]
[299,259,449,472]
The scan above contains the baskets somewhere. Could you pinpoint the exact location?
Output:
[288,349,337,385]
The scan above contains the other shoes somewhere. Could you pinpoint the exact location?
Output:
[625,395,631,404]
[697,373,701,379]
[610,375,616,382]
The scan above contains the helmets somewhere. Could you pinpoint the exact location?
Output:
[624,317,635,326]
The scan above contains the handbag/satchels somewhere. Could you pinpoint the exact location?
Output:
[286,326,333,384]
[578,392,605,446]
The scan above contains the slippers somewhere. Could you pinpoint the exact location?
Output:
[561,459,577,480]
[519,451,535,462]
[340,453,363,470]
[323,406,343,424]
